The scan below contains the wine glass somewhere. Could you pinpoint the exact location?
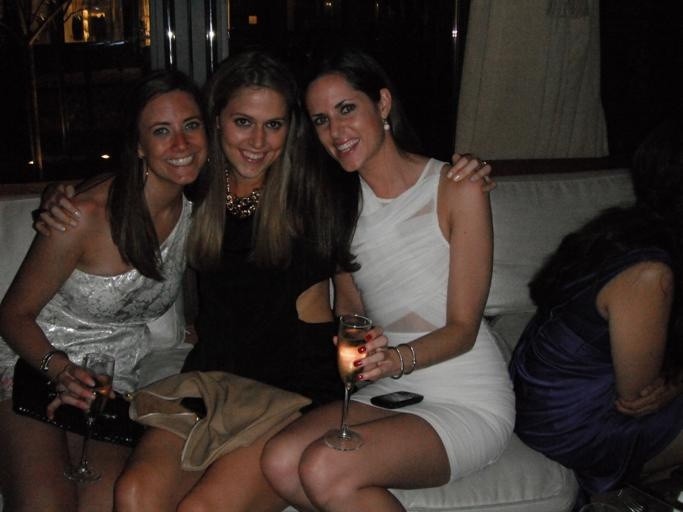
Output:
[62,352,116,483]
[323,312,375,451]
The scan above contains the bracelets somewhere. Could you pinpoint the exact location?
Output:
[397,343,416,375]
[387,344,404,380]
[38,349,68,370]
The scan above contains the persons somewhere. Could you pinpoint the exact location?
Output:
[0,65,215,512]
[260,45,518,512]
[508,114,683,506]
[33,48,496,512]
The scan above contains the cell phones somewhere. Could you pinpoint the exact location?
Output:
[369,390,424,409]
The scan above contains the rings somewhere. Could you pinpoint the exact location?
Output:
[480,160,487,168]
[54,390,65,399]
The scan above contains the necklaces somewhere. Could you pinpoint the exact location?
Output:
[222,167,264,219]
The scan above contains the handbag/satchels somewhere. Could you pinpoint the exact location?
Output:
[126,369,314,471]
[12,356,144,448]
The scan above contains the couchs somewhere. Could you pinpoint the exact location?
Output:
[0,167,636,512]
